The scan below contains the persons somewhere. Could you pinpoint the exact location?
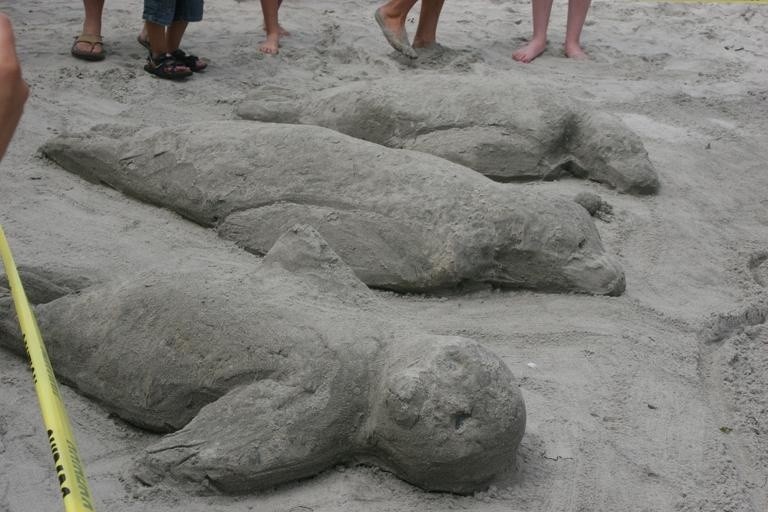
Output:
[143,1,208,79]
[376,1,446,58]
[1,11,30,157]
[258,1,289,55]
[511,0,592,64]
[71,1,152,63]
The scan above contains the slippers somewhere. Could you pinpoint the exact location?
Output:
[71,33,105,61]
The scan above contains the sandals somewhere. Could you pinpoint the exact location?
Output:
[137,35,207,80]
